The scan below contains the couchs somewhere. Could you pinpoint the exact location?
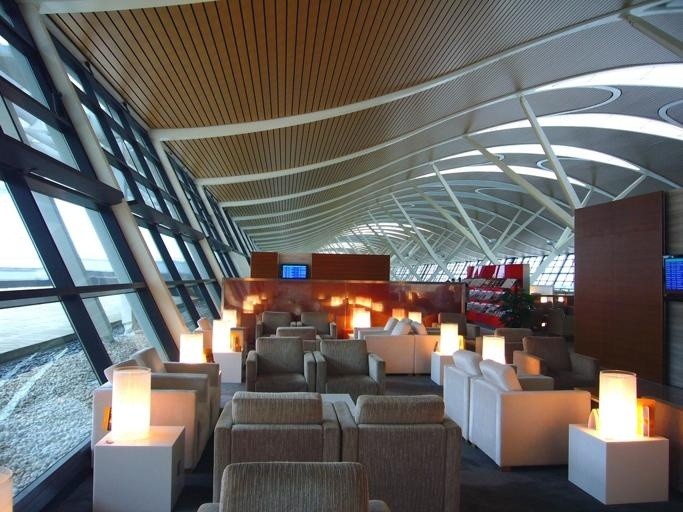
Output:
[92,347,221,470]
[245,336,387,394]
[214,390,461,509]
[192,311,533,383]
[443,336,598,472]
[197,462,388,511]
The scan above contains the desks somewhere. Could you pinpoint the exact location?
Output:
[568,422,669,505]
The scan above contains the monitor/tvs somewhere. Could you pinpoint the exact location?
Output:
[280,264,309,279]
[663,255,682,300]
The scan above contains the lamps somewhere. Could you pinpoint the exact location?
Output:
[599,370,639,440]
[0,466,13,512]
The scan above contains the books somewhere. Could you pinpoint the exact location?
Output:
[464,277,519,318]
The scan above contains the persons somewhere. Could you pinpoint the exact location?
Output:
[446,276,461,283]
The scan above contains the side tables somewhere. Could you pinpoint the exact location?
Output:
[94,424,185,510]
